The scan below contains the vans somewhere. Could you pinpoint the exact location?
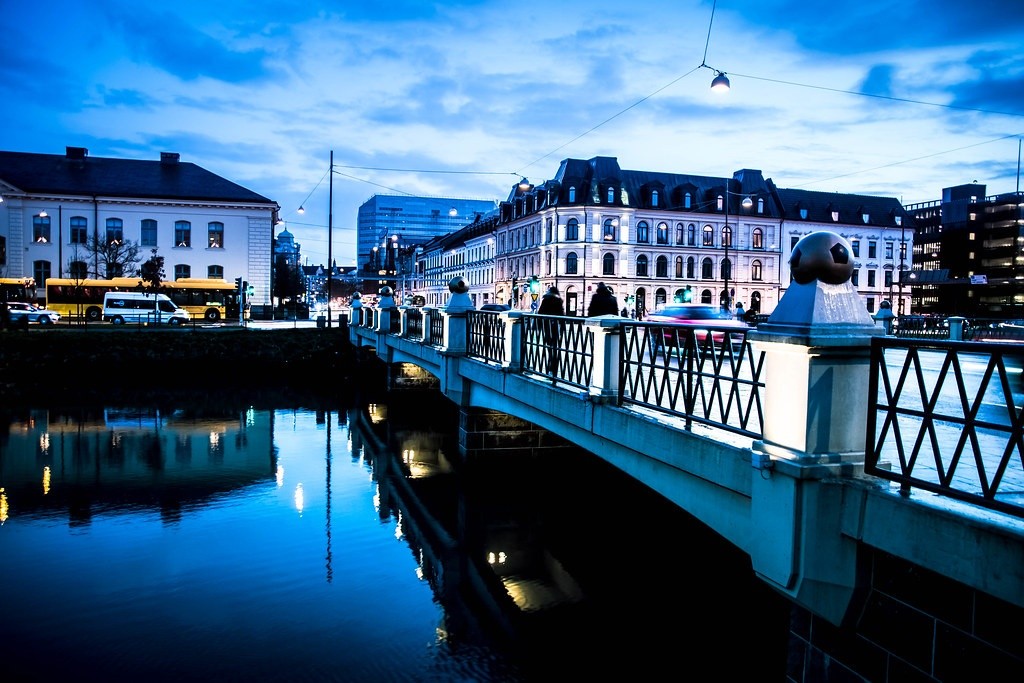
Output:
[102,292,190,326]
[104,409,182,429]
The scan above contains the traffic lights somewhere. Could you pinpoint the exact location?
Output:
[248,286,254,297]
[631,295,634,302]
[624,296,628,303]
[531,280,539,292]
[235,278,240,292]
[685,285,692,300]
[513,278,518,290]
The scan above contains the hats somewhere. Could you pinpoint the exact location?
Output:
[549,287,559,295]
[596,282,607,293]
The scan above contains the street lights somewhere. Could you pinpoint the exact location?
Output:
[40,207,62,278]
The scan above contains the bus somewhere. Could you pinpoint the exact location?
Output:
[0,277,39,309]
[46,276,251,323]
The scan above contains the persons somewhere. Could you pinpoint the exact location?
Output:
[588,282,618,317]
[532,287,566,376]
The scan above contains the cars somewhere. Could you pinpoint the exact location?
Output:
[647,304,749,357]
[7,302,62,326]
[480,304,511,311]
